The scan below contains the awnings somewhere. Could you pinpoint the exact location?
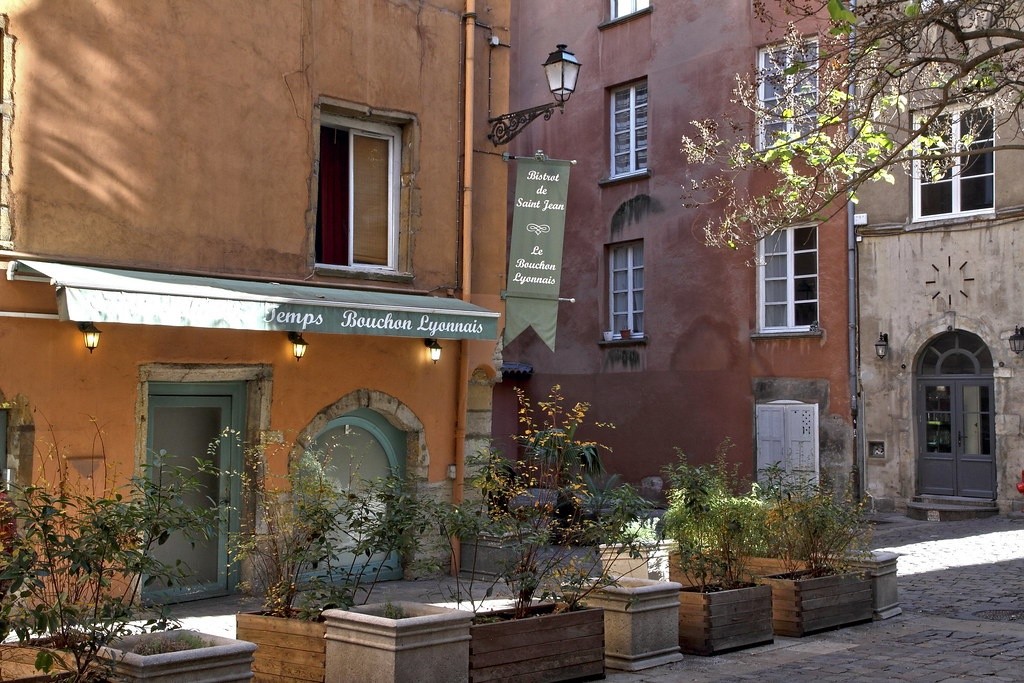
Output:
[15,259,500,341]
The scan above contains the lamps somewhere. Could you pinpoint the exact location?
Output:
[487,43,582,147]
[874,330,888,358]
[1008,324,1024,353]
[77,321,103,354]
[424,337,442,364]
[286,331,309,361]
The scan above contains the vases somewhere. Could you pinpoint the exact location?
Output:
[620,328,630,339]
[603,330,612,340]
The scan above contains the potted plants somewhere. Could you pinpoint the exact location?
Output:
[0,385,902,683]
